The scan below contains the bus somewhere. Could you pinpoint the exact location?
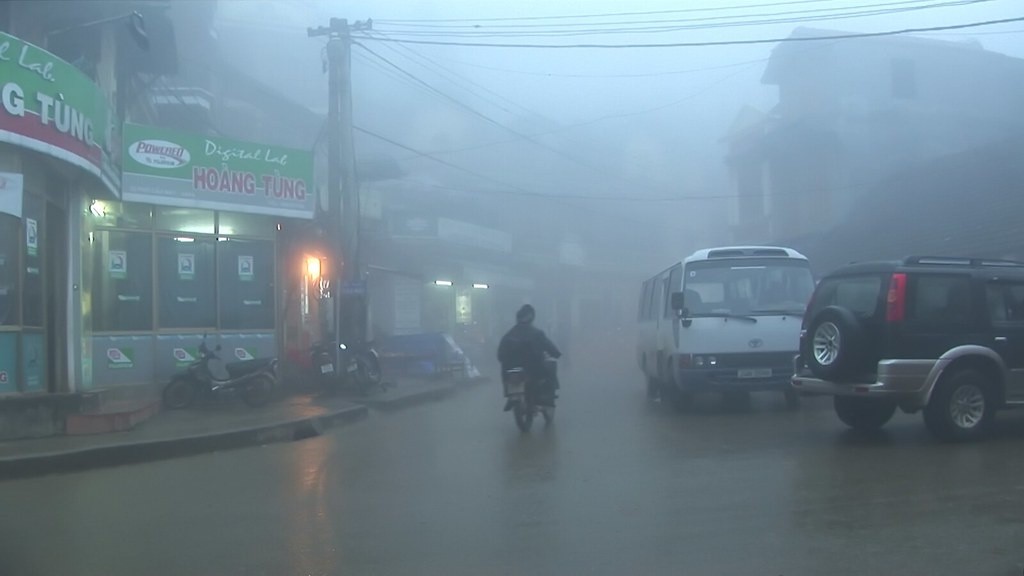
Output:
[634,245,824,417]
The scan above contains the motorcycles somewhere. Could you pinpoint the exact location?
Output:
[159,330,281,406]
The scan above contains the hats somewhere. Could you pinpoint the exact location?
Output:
[517,305,535,323]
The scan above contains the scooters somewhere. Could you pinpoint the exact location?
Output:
[306,337,382,390]
[503,350,559,433]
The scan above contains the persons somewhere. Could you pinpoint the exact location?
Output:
[497,301,558,412]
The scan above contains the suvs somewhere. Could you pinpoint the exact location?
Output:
[788,252,1024,442]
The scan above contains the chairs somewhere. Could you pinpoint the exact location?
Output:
[683,288,702,315]
